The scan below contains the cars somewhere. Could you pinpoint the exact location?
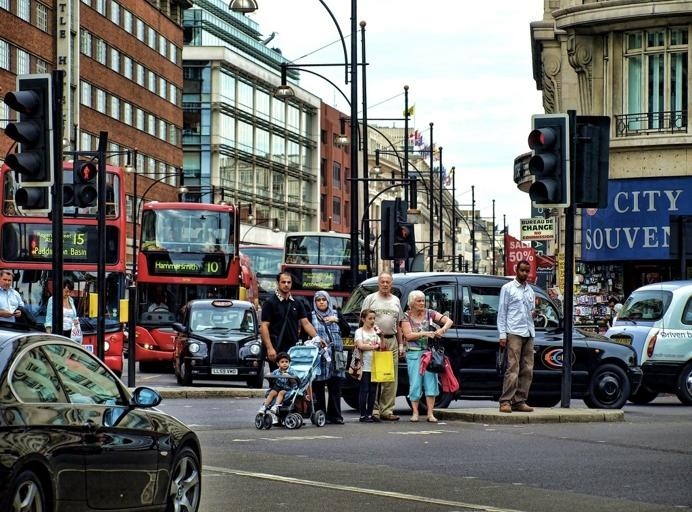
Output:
[122,325,130,359]
[0,329,203,511]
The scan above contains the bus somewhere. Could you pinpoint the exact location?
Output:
[282,230,375,316]
[0,162,127,383]
[131,201,244,372]
[236,244,286,305]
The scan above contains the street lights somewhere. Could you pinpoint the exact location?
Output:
[275,18,372,280]
[374,121,435,273]
[132,165,188,284]
[389,146,512,274]
[334,84,409,212]
[177,184,280,244]
[229,0,360,291]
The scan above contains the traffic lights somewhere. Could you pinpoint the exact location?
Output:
[118,298,128,324]
[83,291,98,319]
[62,183,75,206]
[2,72,54,186]
[528,113,572,208]
[72,159,99,207]
[12,185,52,213]
[394,221,416,260]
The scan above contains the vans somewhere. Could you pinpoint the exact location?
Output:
[171,297,266,388]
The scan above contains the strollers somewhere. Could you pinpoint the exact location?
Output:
[254,343,329,429]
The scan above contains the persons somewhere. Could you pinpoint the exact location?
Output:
[613,281,624,301]
[148,296,168,313]
[44,279,80,338]
[258,271,453,415]
[162,229,178,250]
[197,223,216,242]
[496,260,538,412]
[612,303,623,327]
[0,269,24,331]
[206,289,215,299]
[644,272,657,285]
[608,298,617,315]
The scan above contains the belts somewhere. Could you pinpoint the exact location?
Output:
[384,334,394,338]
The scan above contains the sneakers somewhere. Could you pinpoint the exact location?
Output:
[426,414,437,422]
[327,418,343,424]
[511,401,533,412]
[500,400,512,413]
[257,406,266,414]
[270,404,282,414]
[409,415,419,422]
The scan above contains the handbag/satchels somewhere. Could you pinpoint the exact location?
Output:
[332,350,347,373]
[371,349,394,382]
[426,349,444,373]
[258,335,277,362]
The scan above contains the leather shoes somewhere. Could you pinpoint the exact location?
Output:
[360,414,400,423]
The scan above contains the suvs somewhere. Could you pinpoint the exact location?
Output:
[601,277,692,404]
[330,269,644,411]
